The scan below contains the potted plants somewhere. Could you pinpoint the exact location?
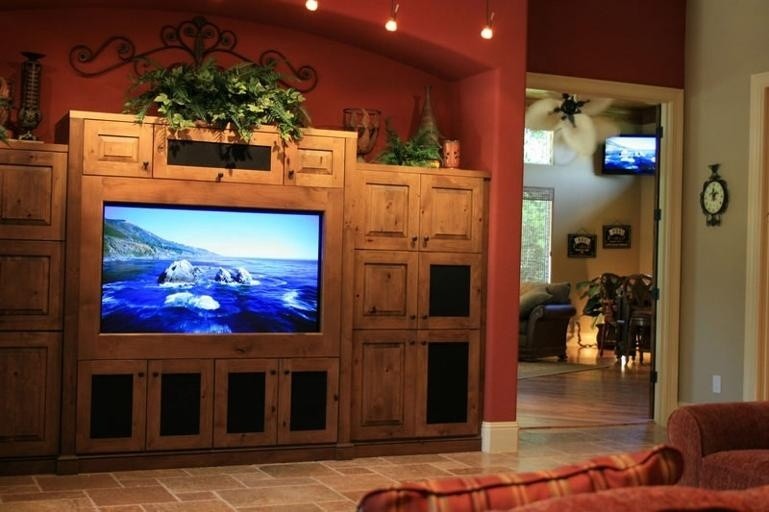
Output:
[575,277,616,350]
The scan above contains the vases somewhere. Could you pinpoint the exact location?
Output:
[344,108,381,163]
[420,84,439,146]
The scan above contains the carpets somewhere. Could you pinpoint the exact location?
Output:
[517,357,609,380]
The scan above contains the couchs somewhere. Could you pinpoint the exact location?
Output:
[509,485,769,512]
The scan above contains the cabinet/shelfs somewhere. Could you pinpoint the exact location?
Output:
[0,110,492,476]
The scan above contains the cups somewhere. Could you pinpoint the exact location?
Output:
[442,138,462,168]
[342,107,382,154]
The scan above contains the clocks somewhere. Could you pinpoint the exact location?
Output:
[699,163,729,227]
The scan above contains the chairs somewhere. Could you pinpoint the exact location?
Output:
[518,304,577,363]
[597,272,653,362]
[667,399,768,490]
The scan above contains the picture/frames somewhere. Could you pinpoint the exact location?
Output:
[568,233,597,258]
[603,224,631,249]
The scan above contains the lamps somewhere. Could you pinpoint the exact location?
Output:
[385,1,400,31]
[304,0,319,12]
[481,12,496,41]
[525,92,614,156]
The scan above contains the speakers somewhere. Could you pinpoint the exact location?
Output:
[91,375,133,438]
[291,371,327,431]
[160,373,200,436]
[227,372,265,433]
[427,342,468,424]
[168,139,271,171]
[430,264,470,317]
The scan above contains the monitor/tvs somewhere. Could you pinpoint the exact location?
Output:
[100,201,322,333]
[602,134,657,175]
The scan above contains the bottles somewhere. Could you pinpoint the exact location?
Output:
[413,82,441,167]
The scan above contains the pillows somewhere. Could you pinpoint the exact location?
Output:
[519,286,554,320]
[355,443,685,512]
[545,282,572,305]
